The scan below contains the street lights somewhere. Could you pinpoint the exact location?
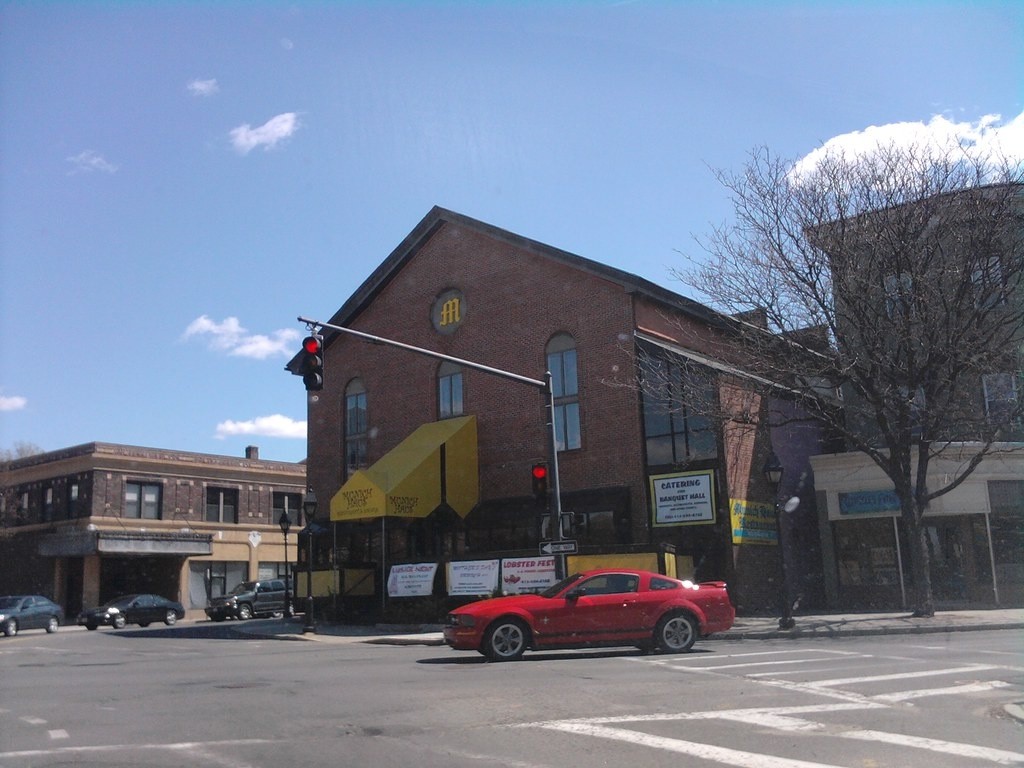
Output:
[303,485,317,631]
[279,510,295,617]
[762,444,796,630]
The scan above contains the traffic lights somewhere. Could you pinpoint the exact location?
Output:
[532,461,547,495]
[302,335,324,391]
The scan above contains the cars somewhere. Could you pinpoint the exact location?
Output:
[203,578,294,621]
[0,596,66,637]
[76,594,186,630]
[444,568,737,662]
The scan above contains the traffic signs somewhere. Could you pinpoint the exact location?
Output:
[539,541,578,556]
